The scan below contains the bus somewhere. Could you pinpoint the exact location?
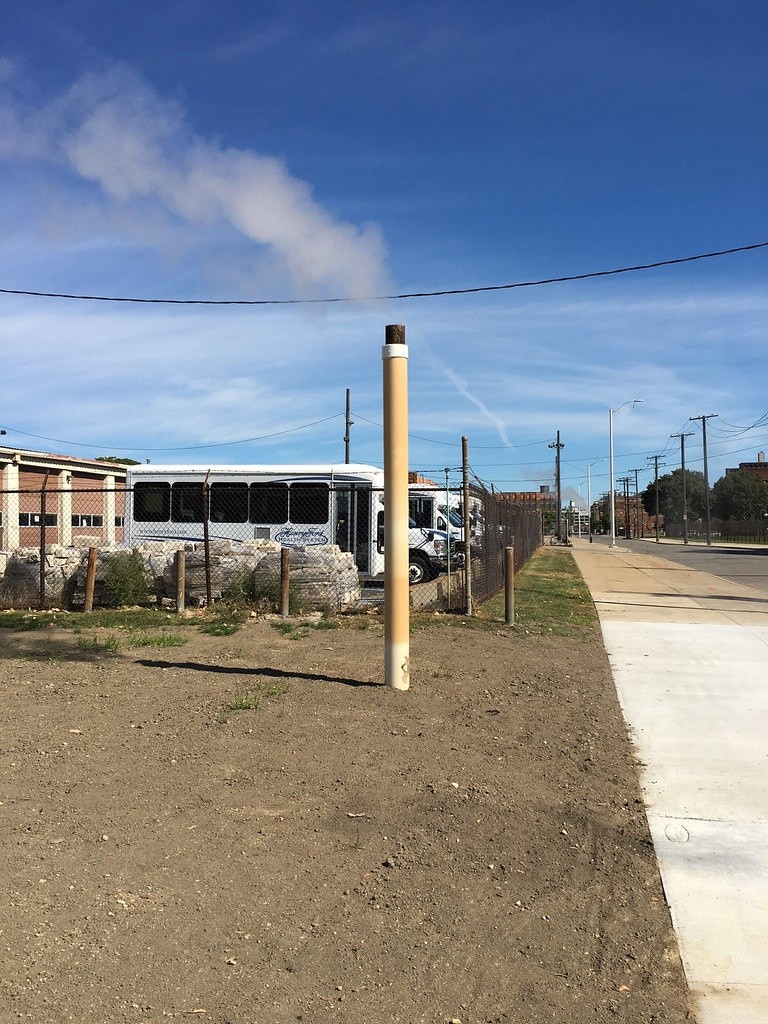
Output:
[124,458,511,587]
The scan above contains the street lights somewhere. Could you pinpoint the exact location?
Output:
[609,399,644,549]
[587,459,606,539]
